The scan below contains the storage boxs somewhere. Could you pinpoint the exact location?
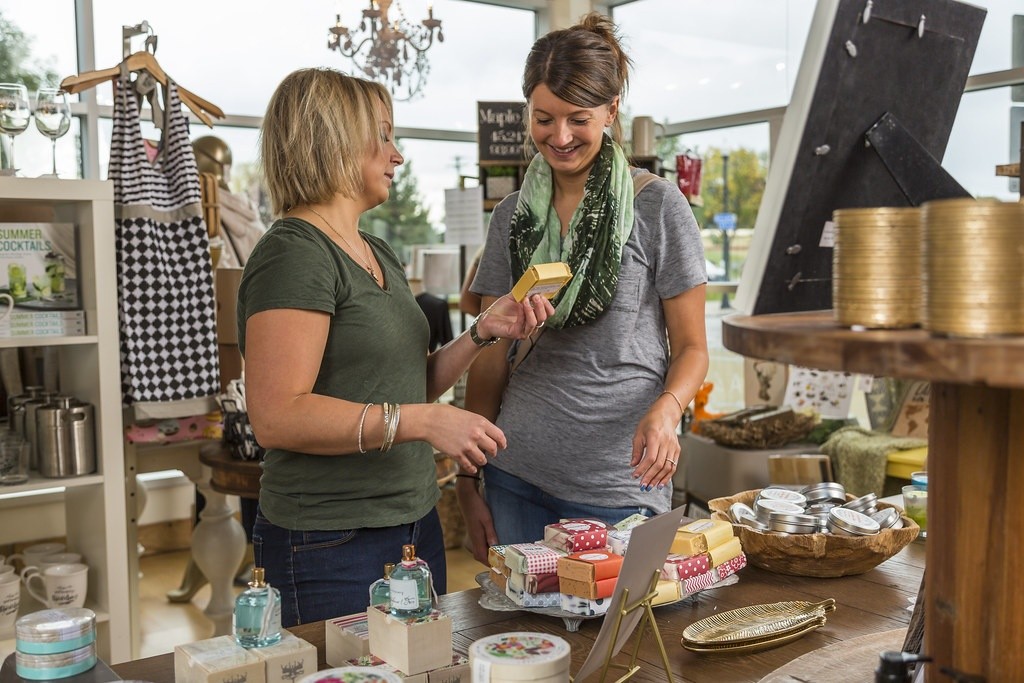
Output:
[216,267,244,345]
[172,602,471,683]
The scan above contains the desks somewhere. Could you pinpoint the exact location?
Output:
[194,440,458,499]
[721,310,1024,683]
[112,541,925,683]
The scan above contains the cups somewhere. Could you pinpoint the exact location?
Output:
[901,469,930,542]
[7,263,27,299]
[6,542,67,588]
[0,553,22,628]
[26,564,91,613]
[21,553,84,599]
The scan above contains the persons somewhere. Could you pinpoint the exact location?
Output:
[456,16,710,569]
[233,67,556,625]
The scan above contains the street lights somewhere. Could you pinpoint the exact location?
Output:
[719,151,732,307]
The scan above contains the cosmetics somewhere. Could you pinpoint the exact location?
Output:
[303,665,402,683]
[15,606,98,680]
[468,631,571,683]
[231,568,282,647]
[368,545,432,619]
[325,611,471,682]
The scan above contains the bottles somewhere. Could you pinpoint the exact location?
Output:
[0,417,33,484]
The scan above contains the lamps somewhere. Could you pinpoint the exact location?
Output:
[325,0,443,103]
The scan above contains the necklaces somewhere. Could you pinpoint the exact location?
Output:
[318,209,385,282]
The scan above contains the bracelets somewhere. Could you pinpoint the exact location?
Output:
[379,401,401,453]
[358,403,374,453]
[657,390,686,416]
[455,474,482,481]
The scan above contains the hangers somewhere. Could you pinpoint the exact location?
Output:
[57,20,227,129]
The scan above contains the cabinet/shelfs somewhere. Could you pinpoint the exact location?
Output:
[1,178,128,667]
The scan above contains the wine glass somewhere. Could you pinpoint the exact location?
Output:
[0,83,31,177]
[36,88,71,178]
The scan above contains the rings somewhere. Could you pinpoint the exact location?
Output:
[536,321,545,328]
[665,456,676,467]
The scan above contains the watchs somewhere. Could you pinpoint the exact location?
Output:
[468,311,500,348]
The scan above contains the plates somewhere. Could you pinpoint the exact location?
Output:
[680,597,838,653]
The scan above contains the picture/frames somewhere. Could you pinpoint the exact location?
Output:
[729,1,986,317]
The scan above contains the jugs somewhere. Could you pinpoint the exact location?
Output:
[32,249,68,294]
